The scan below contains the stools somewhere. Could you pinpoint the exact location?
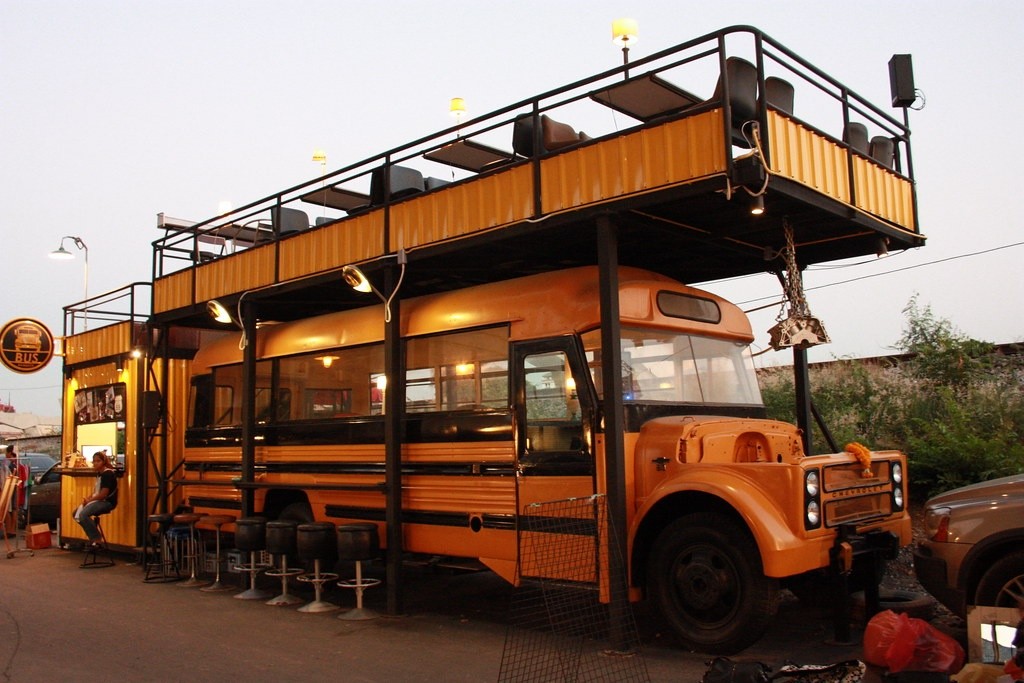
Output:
[335,523,380,617]
[143,513,178,583]
[233,517,272,599]
[78,510,116,569]
[266,518,309,605]
[296,522,340,613]
[201,515,237,592]
[172,513,215,587]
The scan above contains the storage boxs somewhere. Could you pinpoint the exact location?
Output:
[25,522,52,549]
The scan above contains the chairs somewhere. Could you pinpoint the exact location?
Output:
[842,123,896,170]
[707,57,794,131]
[191,109,599,263]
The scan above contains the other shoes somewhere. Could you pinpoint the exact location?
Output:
[86,537,102,549]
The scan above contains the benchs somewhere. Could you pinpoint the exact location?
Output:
[184,410,517,477]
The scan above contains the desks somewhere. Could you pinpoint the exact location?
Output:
[590,72,707,124]
[211,223,266,243]
[423,137,527,175]
[301,184,370,213]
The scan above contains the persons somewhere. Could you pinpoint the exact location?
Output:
[0,445,17,539]
[73,452,117,549]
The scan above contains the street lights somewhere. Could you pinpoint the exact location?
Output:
[48,237,89,332]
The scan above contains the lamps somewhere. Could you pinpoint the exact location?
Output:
[451,97,467,138]
[206,300,244,333]
[749,191,763,215]
[313,147,327,186]
[342,265,387,305]
[314,352,341,369]
[609,17,638,79]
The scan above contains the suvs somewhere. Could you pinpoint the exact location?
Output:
[913,472,1024,621]
[0,452,62,530]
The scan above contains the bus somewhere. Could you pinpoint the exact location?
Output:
[182,266,913,654]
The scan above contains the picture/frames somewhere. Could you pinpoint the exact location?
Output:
[213,385,233,424]
[255,388,290,421]
